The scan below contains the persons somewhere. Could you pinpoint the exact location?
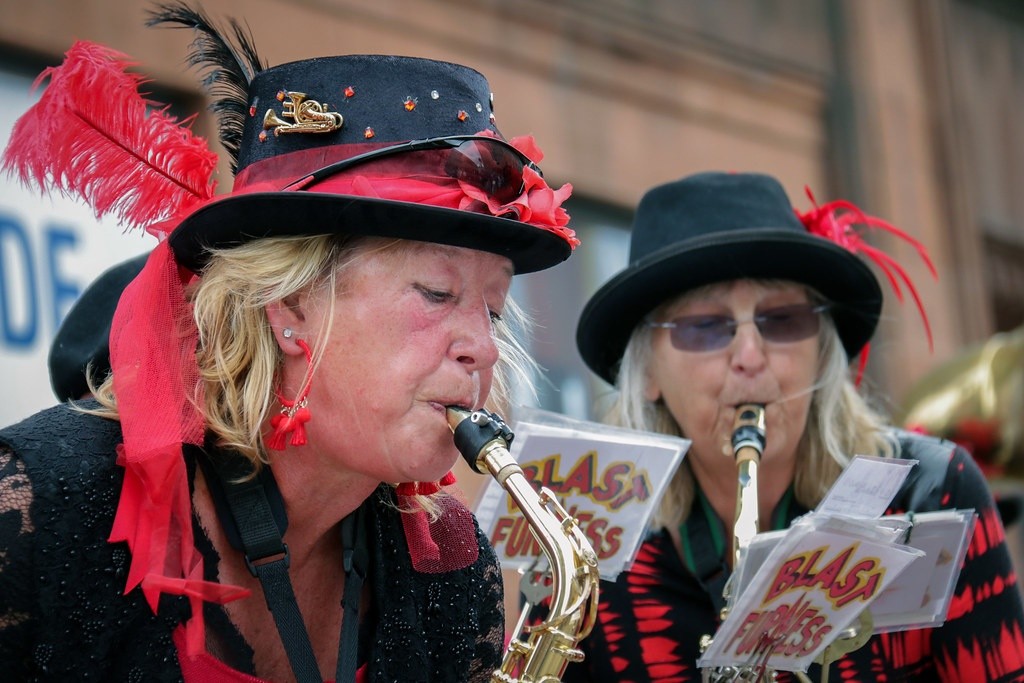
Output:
[0,0,577,683]
[522,167,1024,683]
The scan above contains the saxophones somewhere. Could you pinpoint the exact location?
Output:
[442,402,603,683]
[700,402,777,683]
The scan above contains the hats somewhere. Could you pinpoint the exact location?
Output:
[574,170,940,390]
[48,250,153,404]
[0,0,581,281]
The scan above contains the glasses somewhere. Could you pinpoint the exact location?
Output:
[636,301,833,351]
